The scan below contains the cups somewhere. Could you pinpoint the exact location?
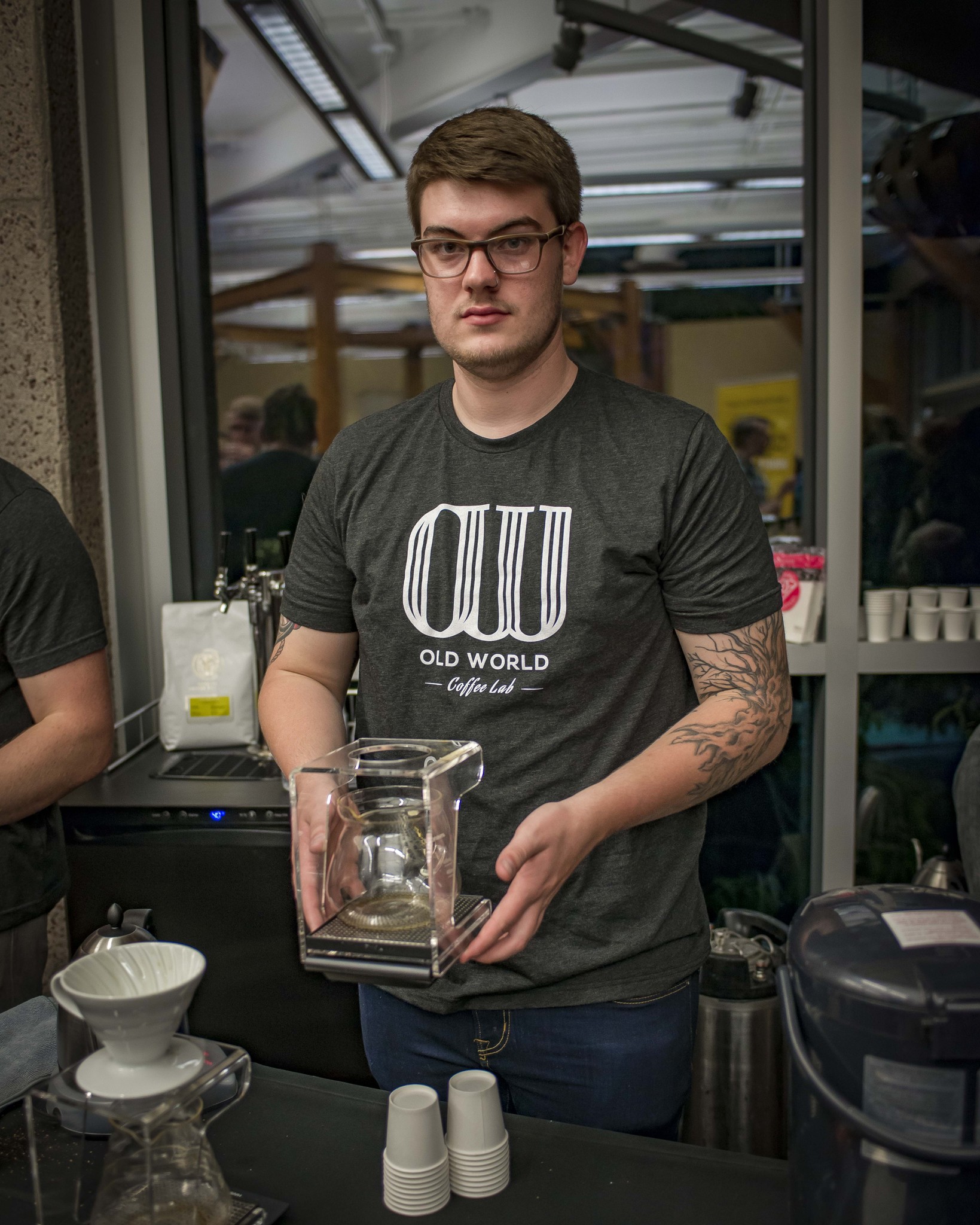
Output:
[383,1084,451,1216]
[864,589,894,643]
[891,588,940,641]
[444,1070,510,1198]
[938,588,980,641]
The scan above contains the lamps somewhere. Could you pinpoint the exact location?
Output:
[730,71,761,120]
[552,18,583,72]
[225,0,403,182]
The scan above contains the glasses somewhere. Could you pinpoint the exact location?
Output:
[410,223,569,279]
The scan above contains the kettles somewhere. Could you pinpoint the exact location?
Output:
[57,903,188,1084]
[911,838,967,893]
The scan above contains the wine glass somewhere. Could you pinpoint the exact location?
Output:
[50,941,206,1099]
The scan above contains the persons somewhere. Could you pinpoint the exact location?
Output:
[219,395,265,476]
[257,104,797,1143]
[766,455,801,526]
[0,455,114,1014]
[729,416,768,507]
[862,404,980,589]
[218,383,320,583]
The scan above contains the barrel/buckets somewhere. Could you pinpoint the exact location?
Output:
[777,883,980,1225]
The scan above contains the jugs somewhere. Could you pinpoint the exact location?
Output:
[88,1088,232,1225]
[326,785,461,930]
